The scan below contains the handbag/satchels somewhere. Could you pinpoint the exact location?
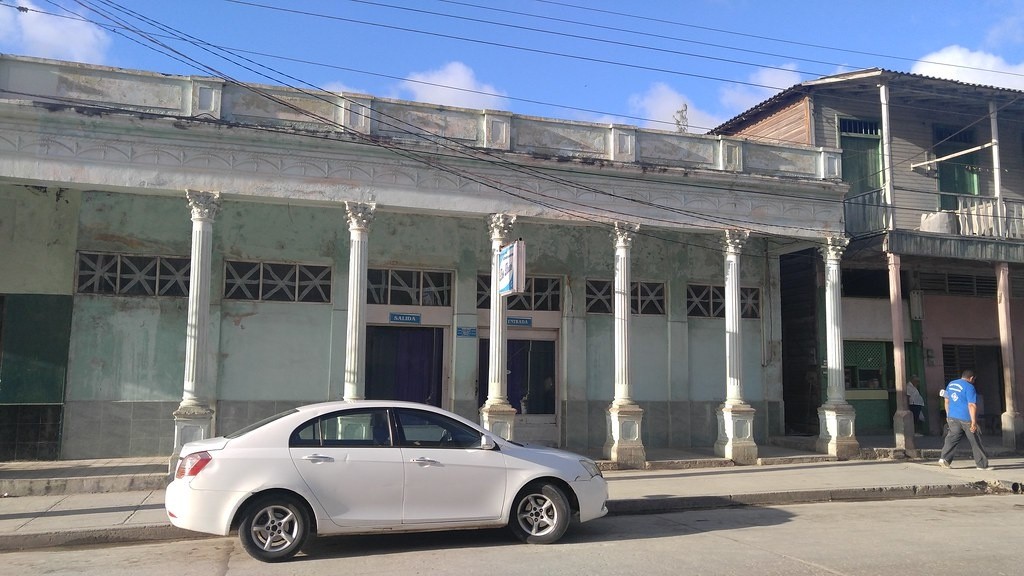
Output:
[919,410,925,421]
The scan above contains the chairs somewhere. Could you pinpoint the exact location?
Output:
[373,423,388,444]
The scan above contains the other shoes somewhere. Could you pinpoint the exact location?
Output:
[977,466,994,470]
[938,458,951,469]
[914,432,924,436]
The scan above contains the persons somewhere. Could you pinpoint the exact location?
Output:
[782,361,794,434]
[905,374,926,437]
[938,368,994,471]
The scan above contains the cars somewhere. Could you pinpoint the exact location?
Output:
[165,399,608,563]
